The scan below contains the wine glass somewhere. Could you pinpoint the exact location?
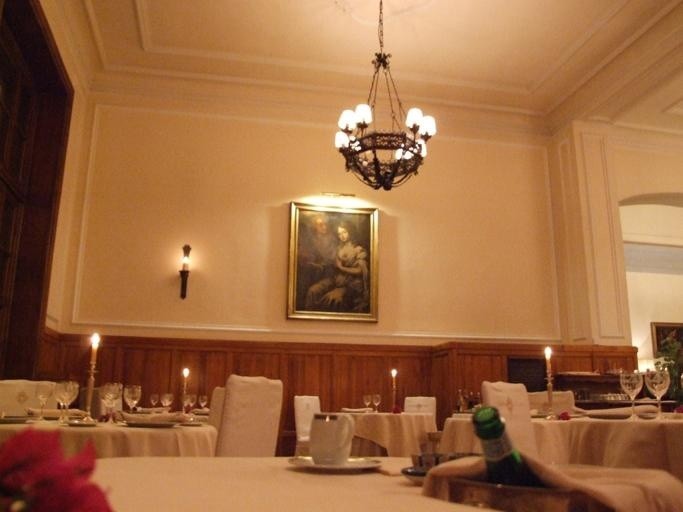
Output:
[362,395,372,413]
[644,365,671,421]
[5,381,209,427]
[372,394,381,413]
[619,368,644,421]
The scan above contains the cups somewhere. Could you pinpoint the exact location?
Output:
[307,411,356,464]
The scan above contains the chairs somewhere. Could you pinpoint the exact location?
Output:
[295,392,323,455]
[211,377,284,459]
[524,392,574,416]
[404,395,440,433]
[482,380,541,458]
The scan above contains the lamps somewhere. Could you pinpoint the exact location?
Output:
[178,244,191,300]
[334,0,437,190]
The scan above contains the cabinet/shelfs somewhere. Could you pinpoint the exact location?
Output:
[429,342,638,432]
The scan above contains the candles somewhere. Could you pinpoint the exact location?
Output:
[544,347,552,371]
[391,369,398,385]
[91,333,100,366]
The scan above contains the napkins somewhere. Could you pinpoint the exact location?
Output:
[24,406,89,419]
[123,413,188,422]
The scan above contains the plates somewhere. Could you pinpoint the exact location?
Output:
[401,465,427,486]
[288,455,385,474]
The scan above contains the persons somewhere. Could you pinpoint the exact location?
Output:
[305,219,369,311]
[297,213,337,272]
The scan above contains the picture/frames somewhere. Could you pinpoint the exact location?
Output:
[286,199,379,323]
[650,321,683,360]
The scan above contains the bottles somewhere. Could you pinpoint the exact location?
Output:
[456,389,483,414]
[471,405,549,489]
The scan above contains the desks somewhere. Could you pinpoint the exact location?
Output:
[572,410,683,482]
